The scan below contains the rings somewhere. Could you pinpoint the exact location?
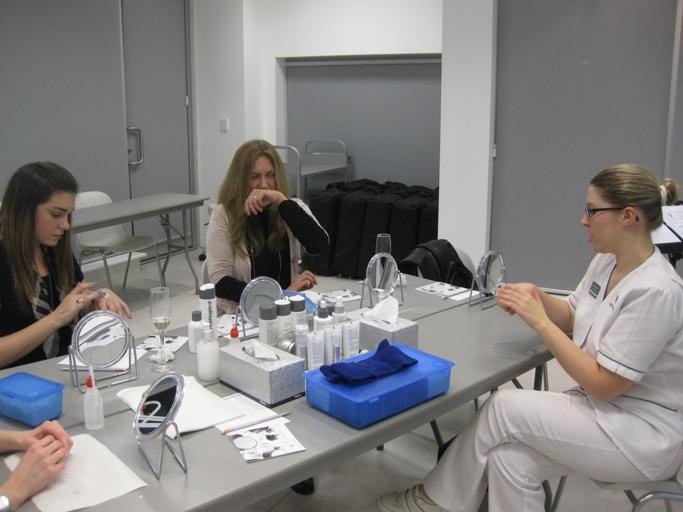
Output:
[251,194,257,199]
[75,298,81,304]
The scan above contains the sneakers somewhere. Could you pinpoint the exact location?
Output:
[375,482,451,512]
[291,477,316,495]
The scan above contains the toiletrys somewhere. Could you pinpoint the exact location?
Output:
[188,282,306,383]
[307,317,361,369]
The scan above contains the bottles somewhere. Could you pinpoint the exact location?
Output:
[195,330,220,380]
[188,311,210,354]
[228,325,241,346]
[83,376,104,429]
[257,294,347,370]
[197,281,217,328]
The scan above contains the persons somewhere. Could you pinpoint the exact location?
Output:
[0,161,133,370]
[0,416,74,512]
[203,140,331,497]
[374,162,683,512]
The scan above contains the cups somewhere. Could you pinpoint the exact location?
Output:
[375,234,391,255]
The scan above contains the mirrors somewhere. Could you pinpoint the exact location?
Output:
[365,252,403,300]
[237,277,282,325]
[477,249,508,297]
[72,307,130,373]
[132,372,183,441]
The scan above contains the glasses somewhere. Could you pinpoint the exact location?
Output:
[584,206,639,222]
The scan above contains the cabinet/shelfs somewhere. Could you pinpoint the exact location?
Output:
[269,137,349,274]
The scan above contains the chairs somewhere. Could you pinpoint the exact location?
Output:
[538,456,682,511]
[372,232,526,456]
[66,187,166,297]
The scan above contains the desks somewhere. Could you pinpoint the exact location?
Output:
[0,259,572,510]
[57,191,211,304]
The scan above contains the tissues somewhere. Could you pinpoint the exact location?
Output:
[215,338,307,407]
[347,294,421,355]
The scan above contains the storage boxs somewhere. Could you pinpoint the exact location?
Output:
[302,341,456,431]
[0,368,65,429]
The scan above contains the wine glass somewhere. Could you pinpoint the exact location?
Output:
[148,287,175,372]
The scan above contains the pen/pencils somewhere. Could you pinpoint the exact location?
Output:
[223,412,291,435]
